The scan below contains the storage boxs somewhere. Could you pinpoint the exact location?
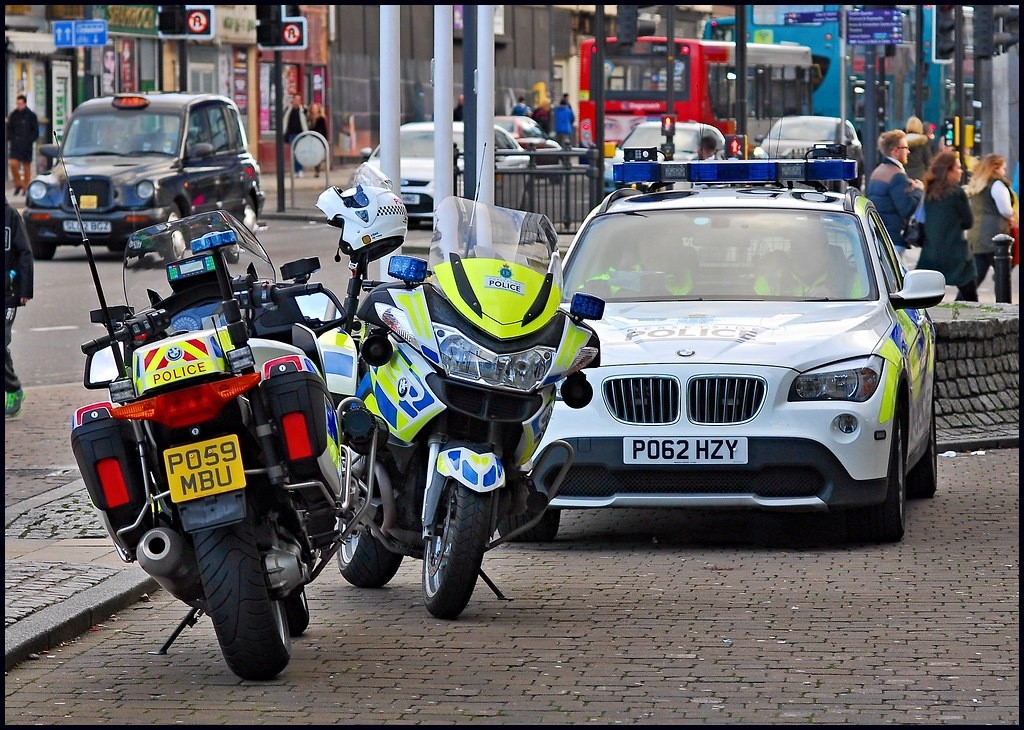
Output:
[258,355,343,506]
[318,327,357,409]
[132,327,237,396]
[70,399,152,553]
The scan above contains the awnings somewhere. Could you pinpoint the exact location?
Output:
[6,29,57,54]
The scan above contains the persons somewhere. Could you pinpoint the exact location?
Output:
[511,93,575,159]
[6,95,39,195]
[5,199,33,414]
[283,95,326,177]
[753,225,861,297]
[868,130,924,256]
[955,154,1014,300]
[692,136,726,188]
[915,151,979,303]
[453,94,464,122]
[903,116,932,180]
[580,222,693,295]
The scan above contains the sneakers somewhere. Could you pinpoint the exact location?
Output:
[5,389,27,418]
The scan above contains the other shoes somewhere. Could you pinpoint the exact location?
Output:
[15,186,22,194]
[22,190,26,196]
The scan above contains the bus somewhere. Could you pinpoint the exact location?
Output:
[701,15,737,45]
[737,4,950,155]
[576,31,823,158]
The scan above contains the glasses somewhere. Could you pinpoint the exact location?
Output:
[891,146,909,152]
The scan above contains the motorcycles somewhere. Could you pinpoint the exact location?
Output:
[317,194,607,619]
[69,210,384,681]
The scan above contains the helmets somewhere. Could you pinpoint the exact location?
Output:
[315,184,407,264]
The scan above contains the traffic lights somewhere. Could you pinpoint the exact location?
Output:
[970,3,1012,59]
[944,117,961,148]
[255,5,308,52]
[154,3,216,41]
[932,3,957,63]
[723,131,740,155]
[1000,4,1021,54]
[617,3,658,46]
[660,115,676,138]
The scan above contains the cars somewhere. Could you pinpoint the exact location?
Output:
[612,120,728,182]
[498,156,948,546]
[352,120,530,223]
[752,115,865,193]
[490,116,566,183]
[21,88,264,263]
